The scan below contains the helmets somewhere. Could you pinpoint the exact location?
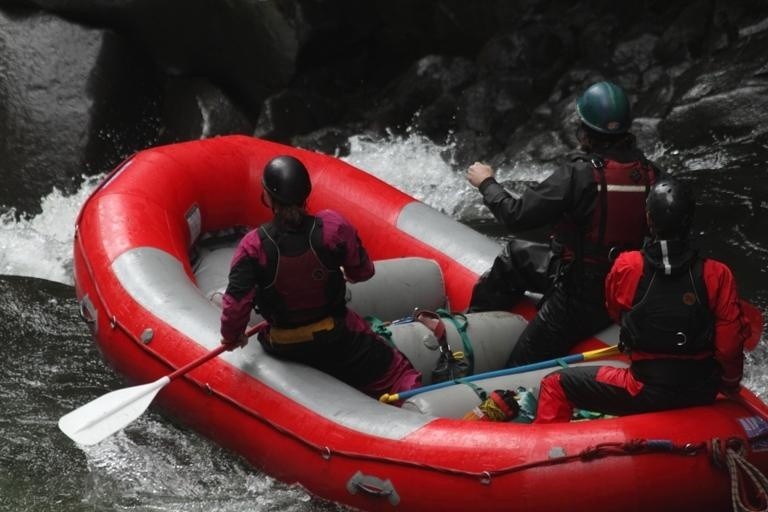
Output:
[260,156,311,203]
[575,80,632,135]
[645,179,695,221]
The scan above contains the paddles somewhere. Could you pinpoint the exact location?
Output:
[58,321,269,446]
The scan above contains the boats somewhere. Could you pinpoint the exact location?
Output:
[73,134,768,512]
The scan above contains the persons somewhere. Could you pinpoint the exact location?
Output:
[466,80,665,369]
[221,155,424,407]
[531,179,745,423]
